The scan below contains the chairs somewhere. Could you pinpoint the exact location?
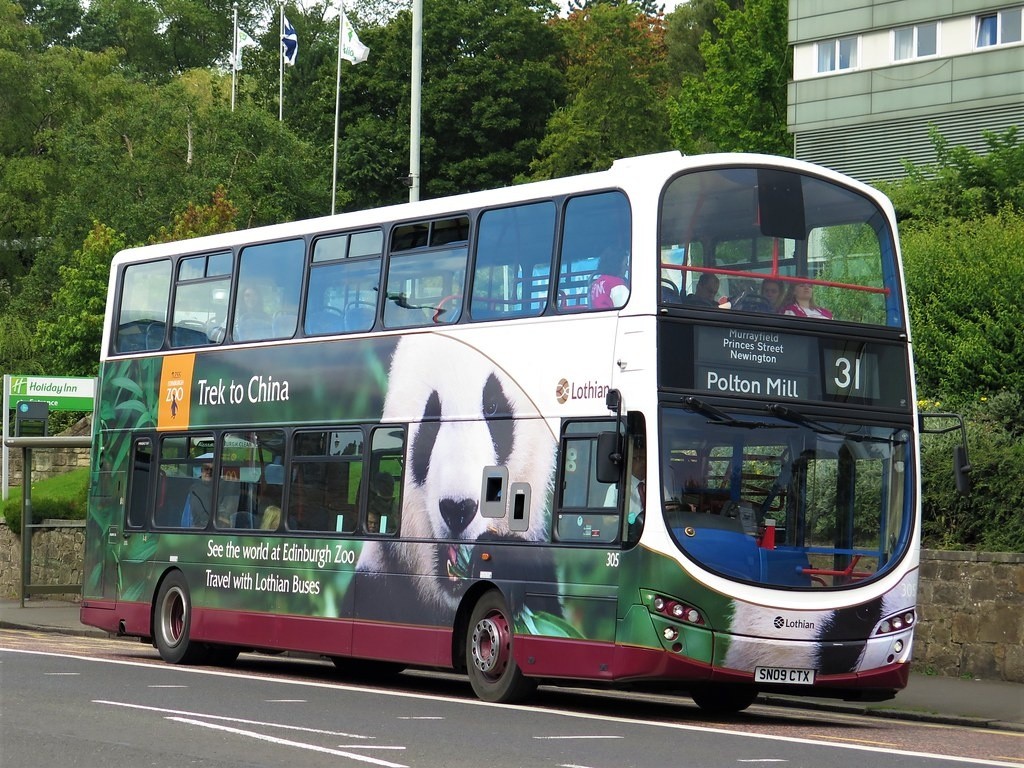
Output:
[119,275,771,350]
[164,475,353,531]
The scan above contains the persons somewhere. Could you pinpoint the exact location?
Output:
[591,244,630,309]
[181,457,236,527]
[261,505,282,529]
[366,507,381,534]
[687,272,834,320]
[370,471,396,531]
[291,439,325,530]
[602,447,696,527]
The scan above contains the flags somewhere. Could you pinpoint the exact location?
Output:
[235,27,258,71]
[281,14,298,67]
[339,8,370,66]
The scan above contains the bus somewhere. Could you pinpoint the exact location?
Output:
[80,149,976,718]
